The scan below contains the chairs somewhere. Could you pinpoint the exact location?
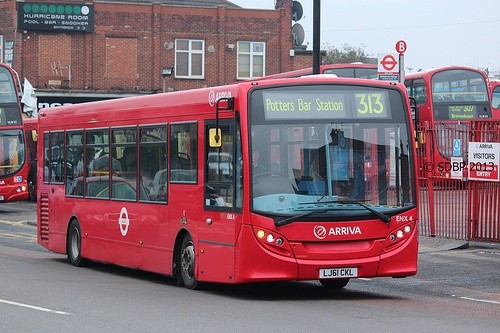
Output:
[171,152,192,170]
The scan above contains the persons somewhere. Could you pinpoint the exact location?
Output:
[252,147,267,184]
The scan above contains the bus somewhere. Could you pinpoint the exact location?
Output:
[232,62,389,187]
[37,78,419,290]
[0,64,31,200]
[490,80,500,130]
[406,67,492,185]
[23,118,70,198]
